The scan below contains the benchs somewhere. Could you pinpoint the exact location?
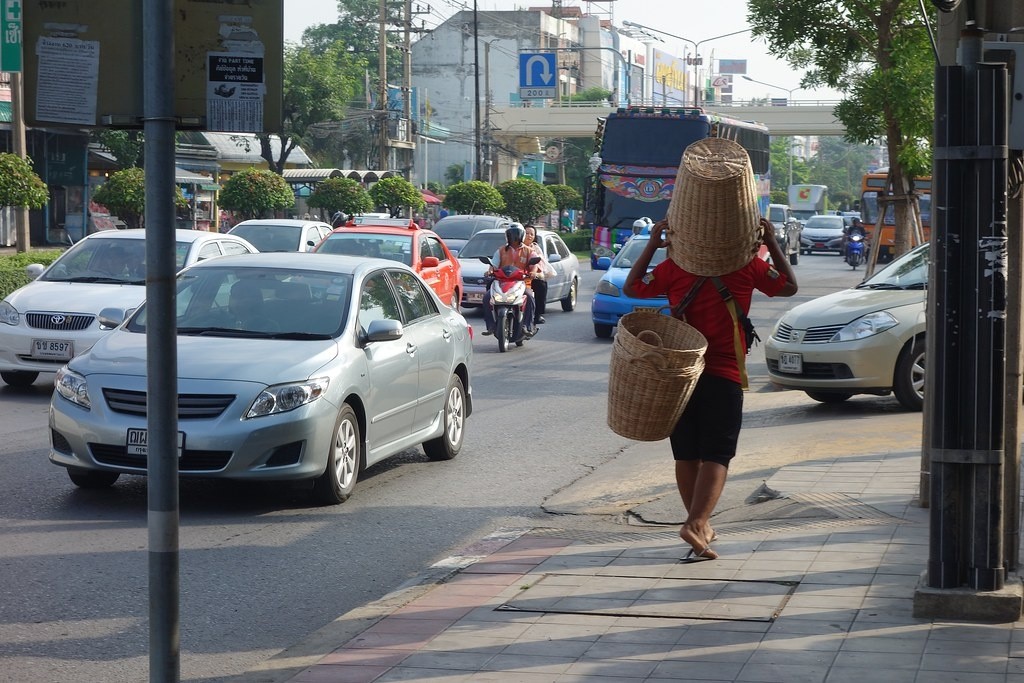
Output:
[262,281,342,334]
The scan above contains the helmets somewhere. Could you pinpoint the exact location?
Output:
[640,217,652,224]
[853,218,860,224]
[504,223,525,242]
[633,220,647,227]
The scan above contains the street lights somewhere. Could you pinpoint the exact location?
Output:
[623,15,769,107]
[740,74,821,184]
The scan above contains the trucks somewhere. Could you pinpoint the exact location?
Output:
[788,183,828,239]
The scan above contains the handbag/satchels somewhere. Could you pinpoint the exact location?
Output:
[745,318,755,354]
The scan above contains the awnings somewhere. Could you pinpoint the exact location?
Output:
[175,165,224,191]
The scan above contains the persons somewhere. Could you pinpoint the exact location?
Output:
[523,224,547,324]
[439,206,450,218]
[481,222,536,335]
[625,217,799,562]
[103,246,130,277]
[628,217,652,238]
[210,280,267,329]
[332,211,349,229]
[845,216,865,260]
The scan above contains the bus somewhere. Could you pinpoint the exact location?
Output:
[858,166,932,263]
[594,107,771,271]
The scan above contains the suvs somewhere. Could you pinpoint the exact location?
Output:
[767,204,799,265]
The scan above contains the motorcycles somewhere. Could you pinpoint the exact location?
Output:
[842,228,872,270]
[479,256,546,352]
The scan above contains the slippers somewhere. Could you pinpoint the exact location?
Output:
[679,546,713,562]
[706,529,718,544]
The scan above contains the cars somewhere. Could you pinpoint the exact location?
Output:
[800,215,849,256]
[1,230,259,388]
[443,230,579,311]
[766,241,927,413]
[432,216,523,258]
[49,253,474,510]
[828,210,862,226]
[224,220,337,256]
[307,218,461,323]
[590,225,692,338]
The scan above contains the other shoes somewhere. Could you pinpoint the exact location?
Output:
[526,331,533,336]
[844,259,847,262]
[538,316,545,323]
[481,329,495,335]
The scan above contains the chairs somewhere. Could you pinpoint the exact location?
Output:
[218,283,265,331]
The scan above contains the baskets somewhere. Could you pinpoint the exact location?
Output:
[665,137,763,277]
[607,306,708,441]
